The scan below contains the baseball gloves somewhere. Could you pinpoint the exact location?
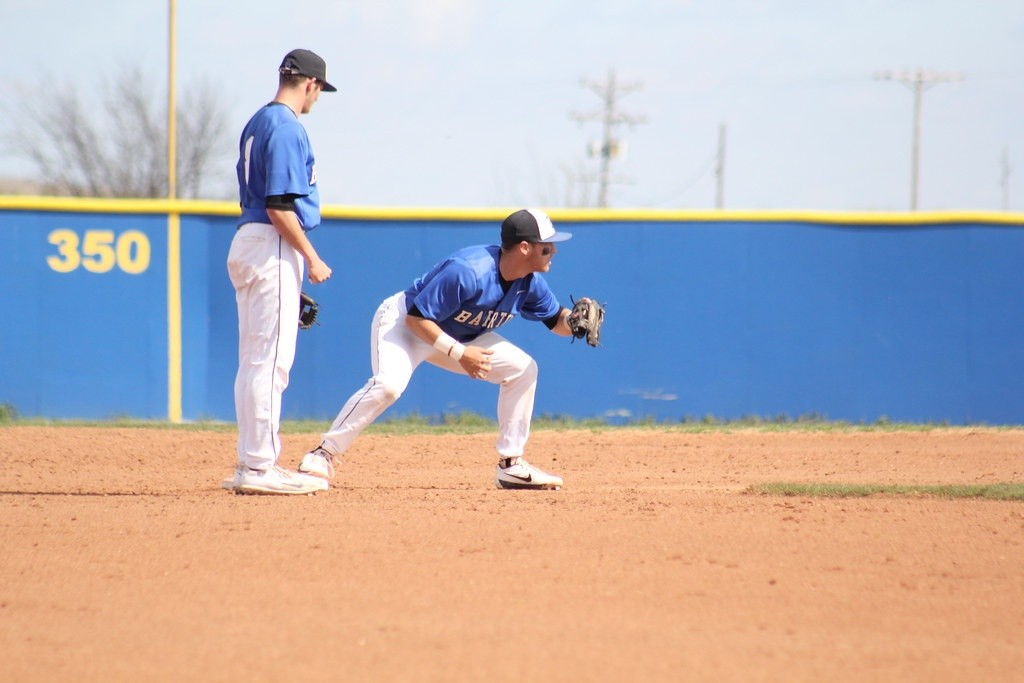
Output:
[297,291,321,330]
[566,294,608,348]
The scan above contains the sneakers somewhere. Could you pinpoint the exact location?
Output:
[300,440,342,479]
[494,457,563,490]
[223,464,328,496]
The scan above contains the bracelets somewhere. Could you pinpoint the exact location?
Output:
[433,331,465,364]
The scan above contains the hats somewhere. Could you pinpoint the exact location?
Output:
[501,208,572,243]
[278,48,337,92]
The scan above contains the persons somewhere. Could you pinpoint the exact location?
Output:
[298,209,607,490]
[226,49,337,495]
